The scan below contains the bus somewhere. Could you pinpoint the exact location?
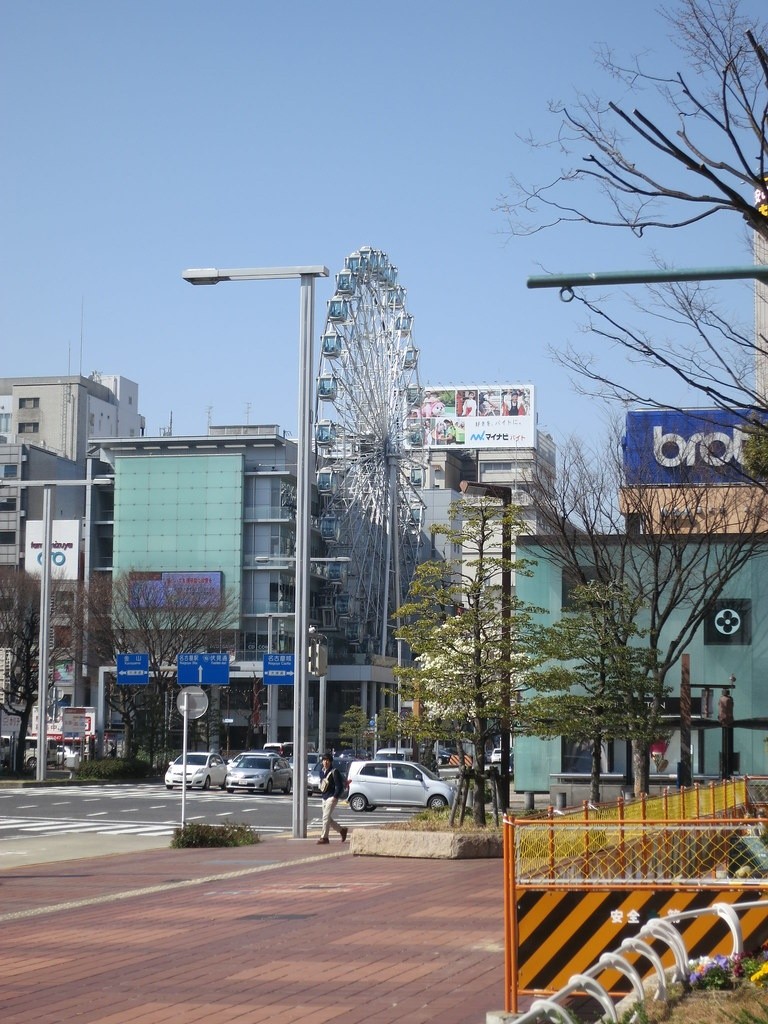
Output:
[262,741,315,758]
[375,748,425,763]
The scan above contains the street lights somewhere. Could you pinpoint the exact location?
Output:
[181,262,330,839]
[456,474,512,815]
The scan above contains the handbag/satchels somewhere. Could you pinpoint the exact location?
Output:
[319,769,336,792]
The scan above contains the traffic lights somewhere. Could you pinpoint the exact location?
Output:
[308,639,329,677]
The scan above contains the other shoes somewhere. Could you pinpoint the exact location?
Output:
[316,838,329,844]
[340,828,348,842]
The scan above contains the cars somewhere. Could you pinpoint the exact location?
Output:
[164,751,227,790]
[225,756,293,795]
[439,749,452,765]
[226,752,280,774]
[289,752,320,774]
[344,759,455,812]
[306,757,359,797]
[0,734,82,769]
[489,747,501,764]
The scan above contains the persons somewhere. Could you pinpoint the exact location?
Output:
[411,391,445,417]
[462,392,476,416]
[406,420,465,445]
[480,389,529,415]
[316,753,348,844]
[107,741,116,757]
